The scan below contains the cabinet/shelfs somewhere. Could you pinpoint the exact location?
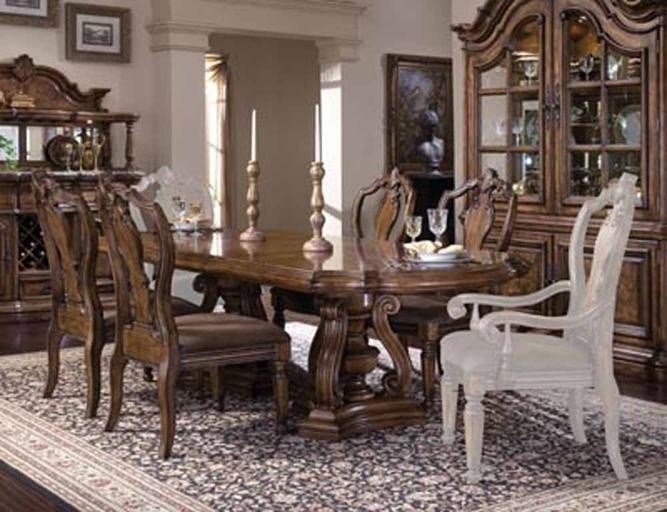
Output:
[448,0,667,215]
[457,215,667,404]
[0,54,145,323]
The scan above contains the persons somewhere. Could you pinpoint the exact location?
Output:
[414,111,444,166]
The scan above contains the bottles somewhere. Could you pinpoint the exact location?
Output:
[580,99,610,197]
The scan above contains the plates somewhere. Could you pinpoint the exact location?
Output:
[613,105,640,143]
[402,255,475,264]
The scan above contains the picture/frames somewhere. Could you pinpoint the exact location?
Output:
[385,53,452,174]
[65,2,132,64]
[0,0,60,29]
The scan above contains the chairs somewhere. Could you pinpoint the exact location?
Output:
[31,169,203,419]
[439,171,638,484]
[352,167,415,245]
[390,169,517,401]
[97,182,291,460]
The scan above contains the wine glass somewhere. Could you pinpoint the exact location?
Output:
[493,116,524,147]
[428,209,448,248]
[405,215,422,243]
[522,62,537,86]
[581,56,595,82]
[170,195,205,237]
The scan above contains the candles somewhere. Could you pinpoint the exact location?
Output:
[251,108,257,160]
[315,103,321,163]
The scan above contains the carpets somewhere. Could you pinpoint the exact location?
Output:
[1,320,667,512]
